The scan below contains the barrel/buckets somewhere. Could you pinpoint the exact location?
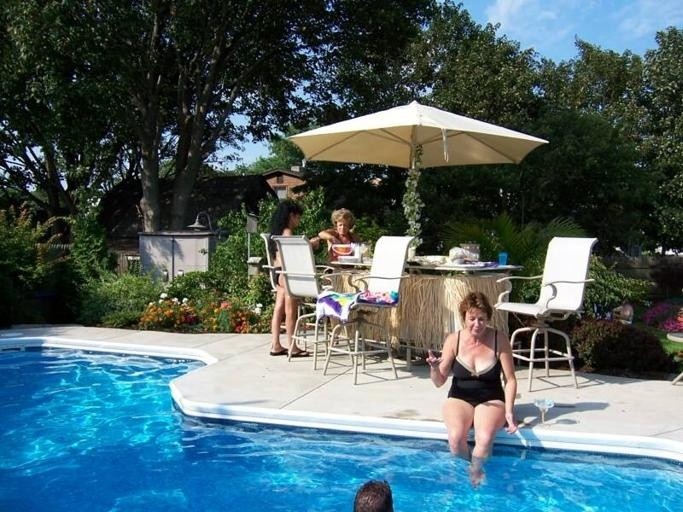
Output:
[331,243,355,269]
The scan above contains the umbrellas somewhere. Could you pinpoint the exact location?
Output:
[284,99,550,247]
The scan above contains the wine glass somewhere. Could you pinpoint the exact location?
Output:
[533,397,555,427]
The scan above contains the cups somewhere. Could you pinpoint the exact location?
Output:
[498,252,508,265]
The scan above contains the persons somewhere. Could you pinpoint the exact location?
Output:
[353,479,394,512]
[268,200,319,358]
[318,207,360,269]
[426,292,518,483]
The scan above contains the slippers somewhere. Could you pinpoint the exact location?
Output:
[270,349,288,356]
[287,350,309,357]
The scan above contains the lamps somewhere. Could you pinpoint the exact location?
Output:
[186,211,213,232]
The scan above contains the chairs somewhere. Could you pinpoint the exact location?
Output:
[259,232,317,351]
[271,235,354,370]
[320,235,415,385]
[493,236,599,392]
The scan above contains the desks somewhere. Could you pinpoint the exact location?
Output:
[327,259,524,373]
